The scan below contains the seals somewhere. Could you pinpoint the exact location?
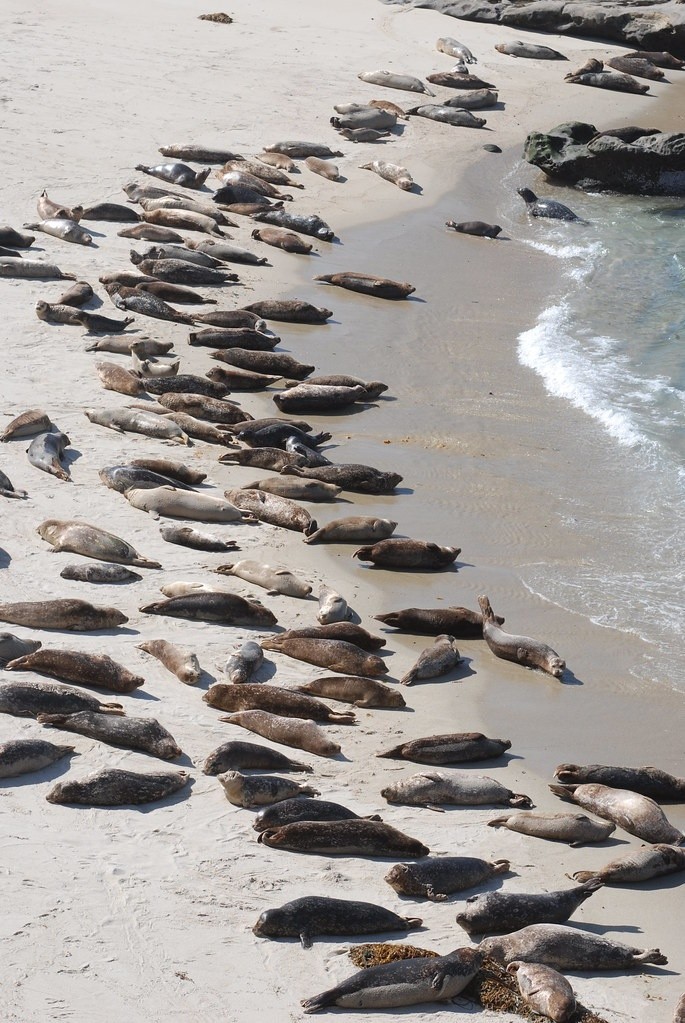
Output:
[0,37,685,1022]
